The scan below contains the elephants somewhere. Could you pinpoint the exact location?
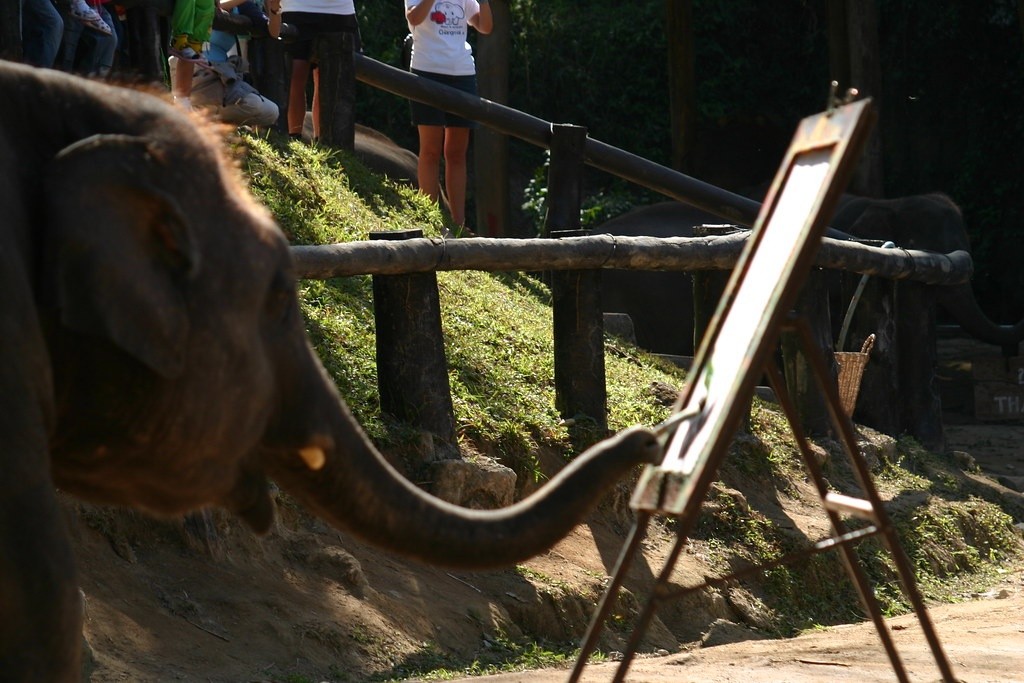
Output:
[0,60,671,683]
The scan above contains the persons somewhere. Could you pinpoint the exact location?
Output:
[280,0,364,144]
[0,0,283,124]
[405,0,494,236]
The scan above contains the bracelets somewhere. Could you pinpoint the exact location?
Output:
[271,5,282,16]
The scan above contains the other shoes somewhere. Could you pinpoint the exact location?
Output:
[441,225,454,238]
